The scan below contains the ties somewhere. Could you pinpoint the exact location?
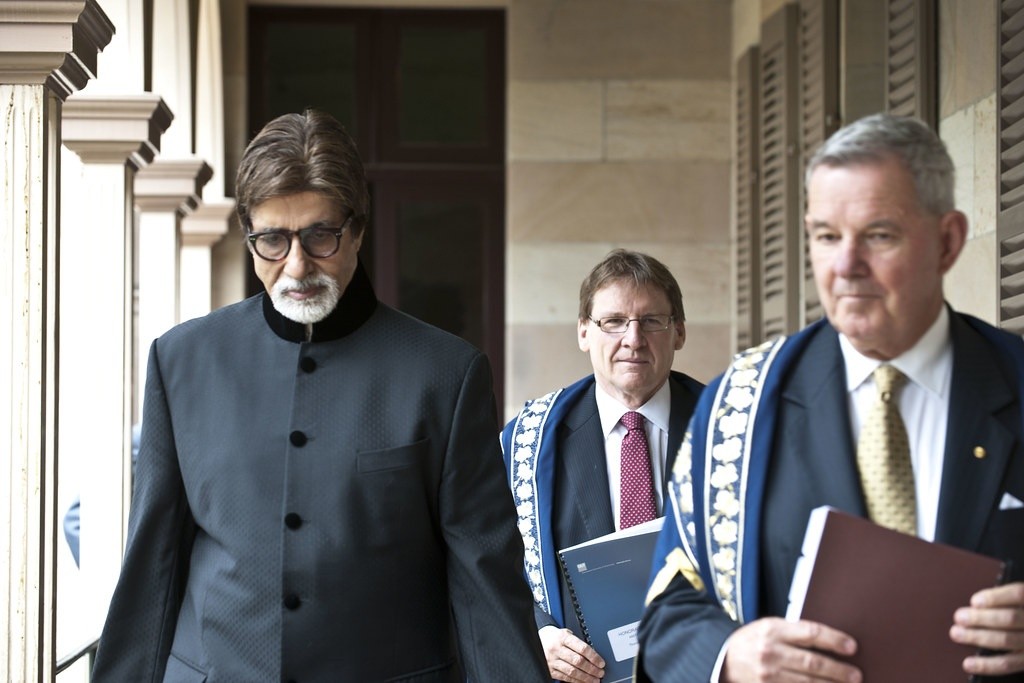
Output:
[619,411,656,529]
[856,365,918,538]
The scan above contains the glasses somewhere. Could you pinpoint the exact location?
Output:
[588,313,674,334]
[248,213,351,262]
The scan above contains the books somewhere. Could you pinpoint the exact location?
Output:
[558,517,665,683]
[784,504,1006,683]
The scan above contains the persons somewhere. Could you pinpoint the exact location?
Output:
[632,112,1024,683]
[497,246,710,683]
[90,107,551,683]
